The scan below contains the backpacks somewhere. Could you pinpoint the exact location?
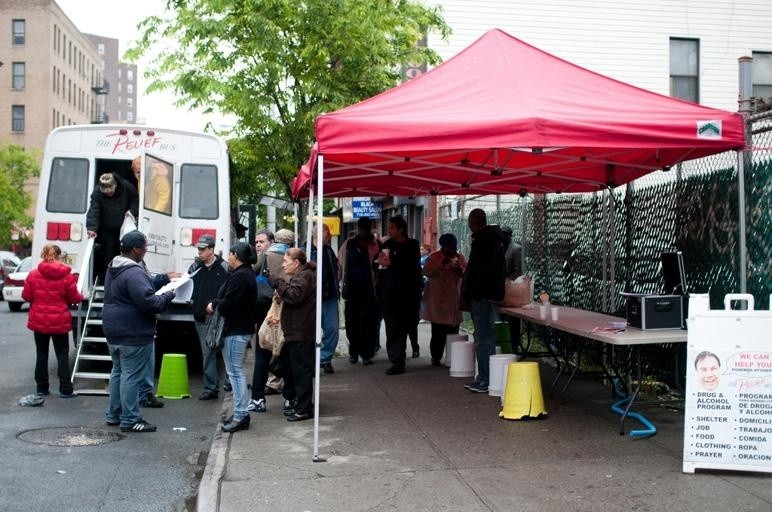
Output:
[256,254,275,322]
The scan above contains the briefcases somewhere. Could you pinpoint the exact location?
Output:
[627,251,688,331]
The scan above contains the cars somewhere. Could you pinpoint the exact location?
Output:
[0,251,22,302]
[3,252,32,313]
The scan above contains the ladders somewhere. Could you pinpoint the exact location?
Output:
[71,275,113,397]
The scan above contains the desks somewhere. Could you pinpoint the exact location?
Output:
[497,301,688,436]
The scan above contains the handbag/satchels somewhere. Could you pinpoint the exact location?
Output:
[258,288,286,357]
[503,277,531,307]
[206,307,225,350]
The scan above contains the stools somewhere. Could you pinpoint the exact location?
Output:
[444,334,468,367]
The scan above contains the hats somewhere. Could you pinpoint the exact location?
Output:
[274,229,295,244]
[196,235,214,248]
[99,173,116,193]
[121,231,154,248]
[501,227,512,233]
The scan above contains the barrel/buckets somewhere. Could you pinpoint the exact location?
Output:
[500,364,508,405]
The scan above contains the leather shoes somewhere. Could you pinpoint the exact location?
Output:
[284,409,296,415]
[287,413,313,421]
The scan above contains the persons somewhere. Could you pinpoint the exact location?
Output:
[695,351,720,390]
[496,226,523,354]
[339,215,424,377]
[301,221,342,374]
[137,260,165,408]
[131,156,172,214]
[457,208,507,392]
[424,234,468,367]
[85,172,140,287]
[100,229,183,434]
[20,243,86,399]
[420,245,432,323]
[187,228,317,432]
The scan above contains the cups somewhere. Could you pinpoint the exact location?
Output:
[552,308,559,320]
[540,306,549,318]
[383,249,390,261]
[452,257,459,268]
[543,303,551,315]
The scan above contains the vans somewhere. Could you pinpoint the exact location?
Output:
[30,123,231,347]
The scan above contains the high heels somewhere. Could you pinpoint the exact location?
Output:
[221,414,250,432]
[223,415,233,425]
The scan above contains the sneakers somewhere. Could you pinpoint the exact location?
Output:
[412,344,419,358]
[470,383,488,392]
[37,391,50,396]
[107,421,120,425]
[199,391,218,400]
[349,355,358,364]
[431,358,440,366]
[120,420,156,432]
[60,393,77,398]
[464,382,478,388]
[283,400,294,409]
[320,363,334,374]
[145,393,163,408]
[248,399,266,411]
[264,387,279,394]
[385,365,405,375]
[223,383,232,391]
[363,358,371,365]
